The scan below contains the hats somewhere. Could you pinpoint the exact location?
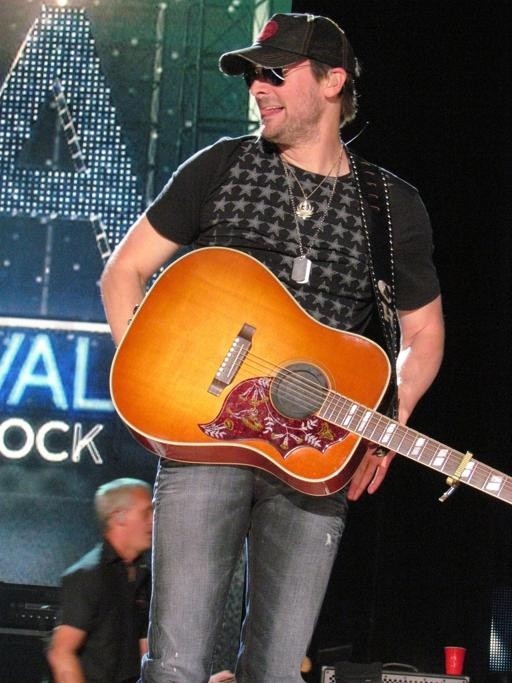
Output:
[217,13,359,77]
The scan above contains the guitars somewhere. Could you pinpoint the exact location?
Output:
[108,247,512,504]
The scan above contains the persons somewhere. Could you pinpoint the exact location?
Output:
[43,476,154,682]
[98,8,451,682]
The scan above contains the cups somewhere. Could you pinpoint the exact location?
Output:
[444,646,466,676]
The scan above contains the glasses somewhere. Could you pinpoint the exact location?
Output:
[243,65,303,86]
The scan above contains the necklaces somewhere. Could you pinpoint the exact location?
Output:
[281,145,341,221]
[274,150,340,285]
